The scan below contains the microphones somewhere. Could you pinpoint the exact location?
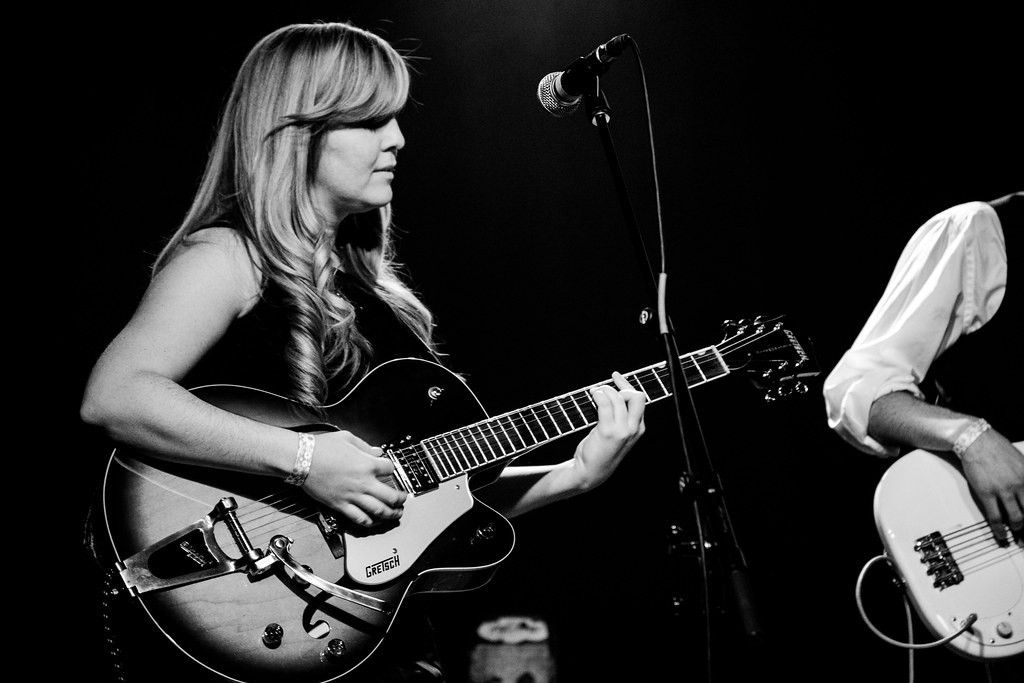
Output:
[536,33,629,119]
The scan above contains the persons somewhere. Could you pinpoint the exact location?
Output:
[822,192,1024,683]
[80,22,647,683]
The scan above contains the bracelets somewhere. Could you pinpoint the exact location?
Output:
[285,431,315,485]
[952,419,991,457]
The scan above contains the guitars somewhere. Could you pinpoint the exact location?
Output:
[871,439,1024,661]
[90,314,826,683]
